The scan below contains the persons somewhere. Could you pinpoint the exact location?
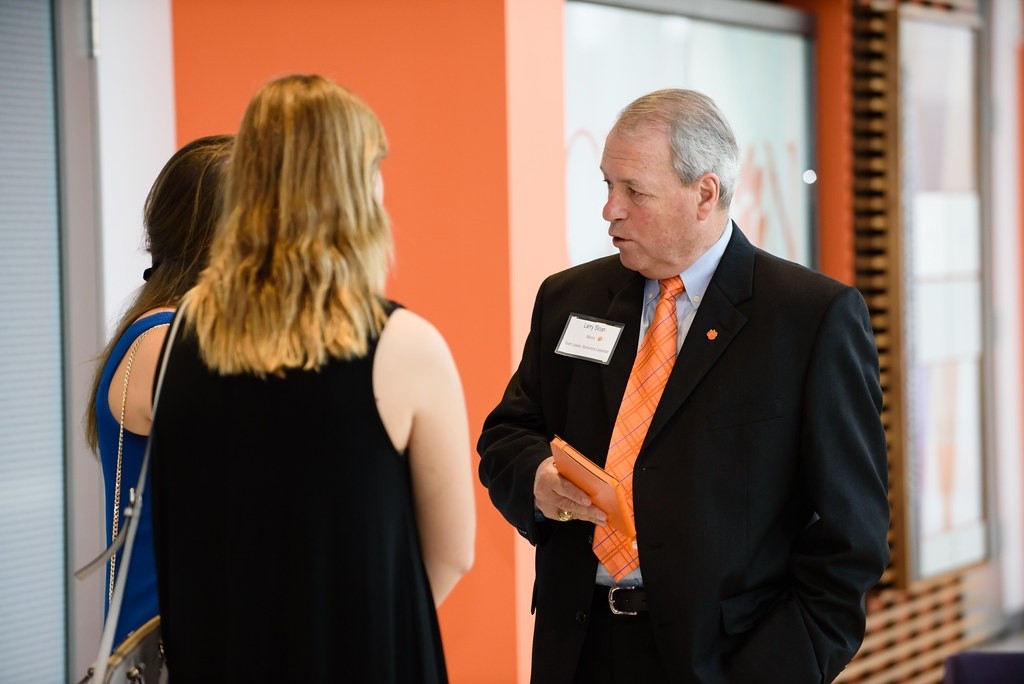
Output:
[77,71,476,684]
[476,90,891,684]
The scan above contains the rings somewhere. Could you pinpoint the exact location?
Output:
[557,508,571,522]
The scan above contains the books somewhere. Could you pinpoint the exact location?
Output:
[550,435,637,538]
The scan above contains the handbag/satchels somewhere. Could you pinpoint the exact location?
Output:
[87,614,169,684]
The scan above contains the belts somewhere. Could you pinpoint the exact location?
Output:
[596,583,655,619]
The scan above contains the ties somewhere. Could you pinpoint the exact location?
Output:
[591,275,687,583]
